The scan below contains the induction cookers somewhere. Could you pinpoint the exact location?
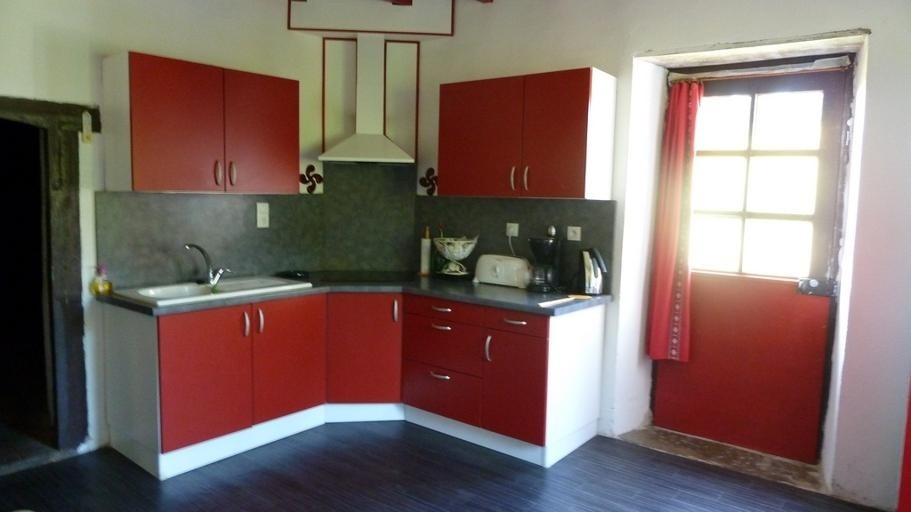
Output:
[303,270,422,287]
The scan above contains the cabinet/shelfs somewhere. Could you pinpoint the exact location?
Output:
[328,292,404,422]
[100,292,328,483]
[438,66,618,201]
[99,50,300,197]
[403,294,486,468]
[482,303,605,470]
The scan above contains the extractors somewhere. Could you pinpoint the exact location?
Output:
[318,33,415,163]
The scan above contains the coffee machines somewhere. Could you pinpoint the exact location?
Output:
[527,238,559,292]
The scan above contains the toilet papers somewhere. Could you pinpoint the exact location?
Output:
[421,237,432,275]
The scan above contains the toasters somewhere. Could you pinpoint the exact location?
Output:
[472,253,531,290]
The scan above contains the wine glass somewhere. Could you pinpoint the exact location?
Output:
[433,237,478,276]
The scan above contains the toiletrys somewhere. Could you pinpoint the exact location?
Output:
[91,264,113,300]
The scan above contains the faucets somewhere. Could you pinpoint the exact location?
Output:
[184,242,232,287]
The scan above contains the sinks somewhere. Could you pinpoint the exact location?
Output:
[113,281,214,307]
[214,275,313,300]
